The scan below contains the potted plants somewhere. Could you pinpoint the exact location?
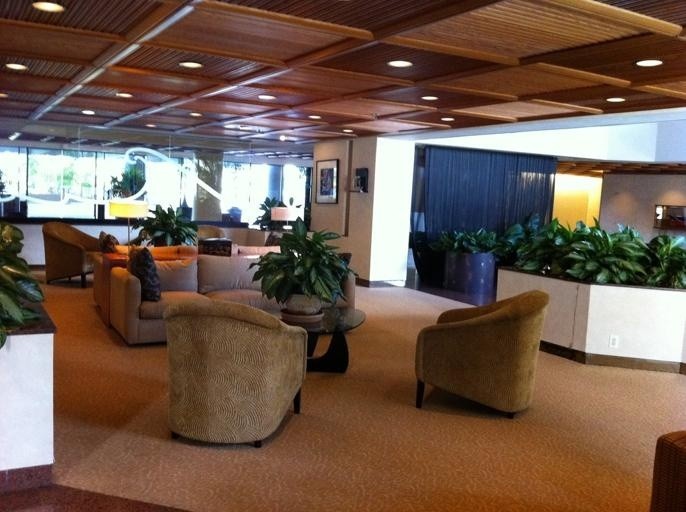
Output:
[247,216,359,315]
[0,219,56,472]
[427,227,510,290]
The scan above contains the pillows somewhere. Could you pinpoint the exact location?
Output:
[127,244,161,301]
[263,232,281,246]
[335,252,352,266]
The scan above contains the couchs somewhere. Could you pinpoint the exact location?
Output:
[109,252,357,347]
[159,298,309,449]
[87,244,283,326]
[39,220,105,289]
[413,289,549,418]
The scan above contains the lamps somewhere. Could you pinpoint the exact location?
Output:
[109,200,148,254]
[270,206,304,234]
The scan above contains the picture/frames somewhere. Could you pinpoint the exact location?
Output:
[314,158,339,205]
